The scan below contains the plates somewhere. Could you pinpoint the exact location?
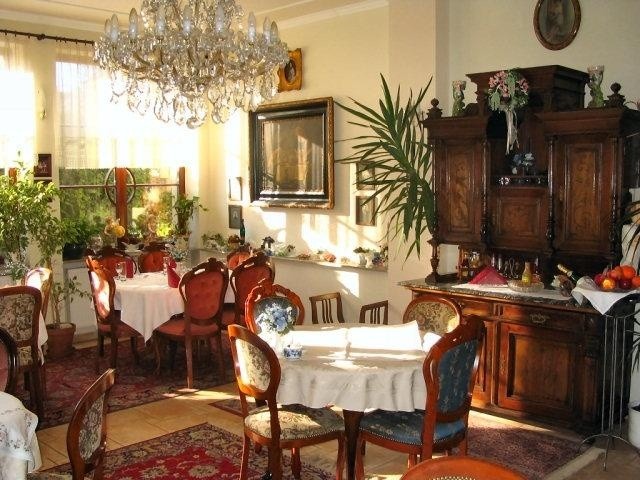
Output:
[604,287,638,293]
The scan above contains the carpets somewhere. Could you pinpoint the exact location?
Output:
[429,414,606,480]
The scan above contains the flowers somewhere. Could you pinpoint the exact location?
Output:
[487,68,530,114]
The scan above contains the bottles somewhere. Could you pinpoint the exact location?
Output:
[524,153,538,183]
[510,153,523,183]
[557,264,601,291]
[456,249,541,288]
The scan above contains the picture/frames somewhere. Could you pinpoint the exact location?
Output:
[34,180,54,202]
[228,204,243,229]
[228,176,243,202]
[356,160,377,191]
[355,195,376,227]
[277,47,303,93]
[34,153,53,178]
[247,96,335,210]
[533,0,581,51]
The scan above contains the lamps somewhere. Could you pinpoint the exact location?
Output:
[91,0,290,129]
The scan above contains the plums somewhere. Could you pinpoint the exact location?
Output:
[594,274,605,286]
[620,280,631,290]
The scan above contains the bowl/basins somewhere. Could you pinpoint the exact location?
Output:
[506,279,545,291]
[283,345,302,361]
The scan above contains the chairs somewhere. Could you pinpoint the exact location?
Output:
[27,368,114,480]
[220,242,275,331]
[1,268,53,421]
[227,324,346,479]
[152,257,229,389]
[309,292,346,324]
[359,300,389,324]
[86,247,167,385]
[244,278,305,334]
[354,314,488,480]
[403,294,463,336]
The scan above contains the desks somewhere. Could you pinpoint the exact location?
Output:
[1,391,42,479]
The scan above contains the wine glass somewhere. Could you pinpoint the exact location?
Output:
[115,263,123,281]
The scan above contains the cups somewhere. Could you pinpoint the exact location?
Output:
[164,256,219,278]
[119,262,127,282]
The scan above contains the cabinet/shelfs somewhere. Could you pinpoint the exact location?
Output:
[420,64,640,259]
[396,274,636,444]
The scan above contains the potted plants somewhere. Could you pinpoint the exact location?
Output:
[62,215,100,260]
[170,195,210,250]
[0,151,95,359]
